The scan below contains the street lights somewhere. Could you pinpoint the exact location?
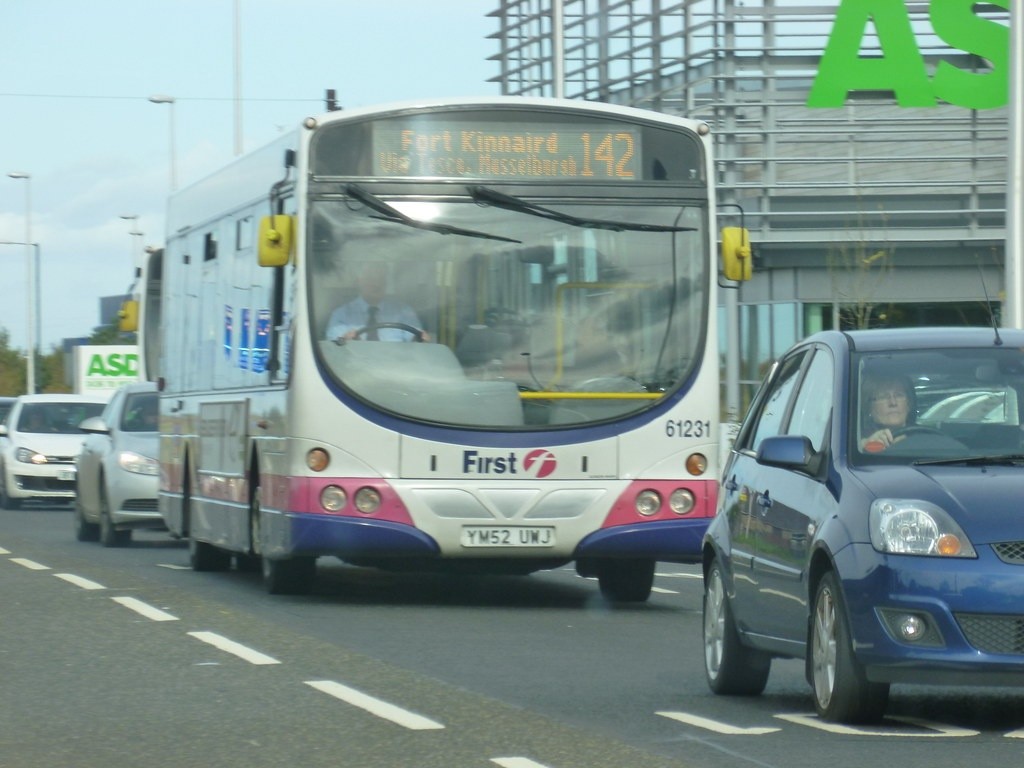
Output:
[120,212,144,270]
[9,170,36,395]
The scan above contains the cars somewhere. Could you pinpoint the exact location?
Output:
[75,383,187,546]
[1,394,110,510]
[702,322,1024,726]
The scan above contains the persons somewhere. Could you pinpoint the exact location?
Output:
[858,374,919,456]
[328,258,433,344]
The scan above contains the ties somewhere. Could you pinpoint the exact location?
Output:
[365,307,379,341]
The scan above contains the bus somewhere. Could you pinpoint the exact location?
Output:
[159,96,752,605]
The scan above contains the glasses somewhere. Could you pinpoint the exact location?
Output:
[870,391,909,402]
